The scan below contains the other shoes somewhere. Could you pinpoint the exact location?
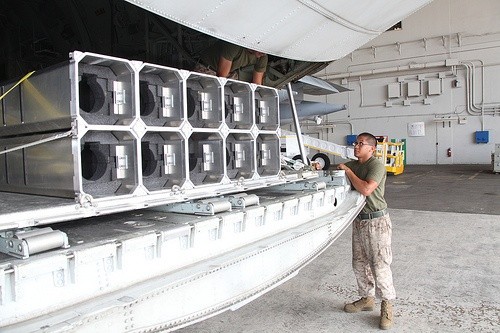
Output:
[379,299,393,330]
[344,296,375,313]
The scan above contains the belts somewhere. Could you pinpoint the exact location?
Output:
[194,56,217,73]
[357,208,387,219]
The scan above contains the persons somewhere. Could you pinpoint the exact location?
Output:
[311,132,396,330]
[193,41,269,86]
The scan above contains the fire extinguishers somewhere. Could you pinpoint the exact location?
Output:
[448,148,451,157]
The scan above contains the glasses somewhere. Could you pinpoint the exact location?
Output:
[352,142,372,147]
